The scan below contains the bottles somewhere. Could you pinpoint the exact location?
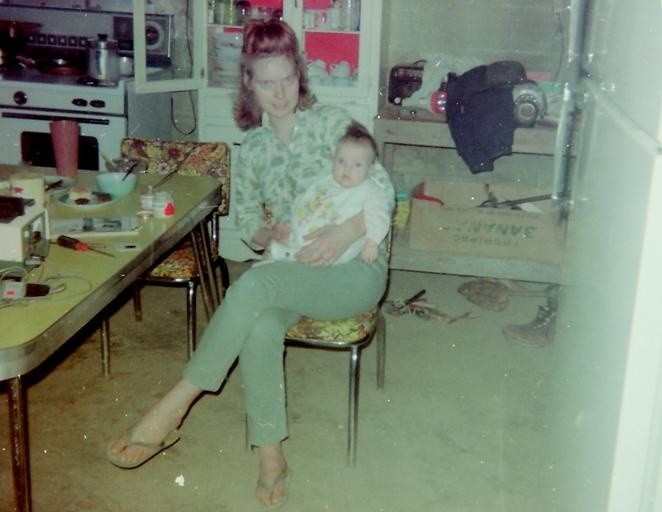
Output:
[84,31,120,88]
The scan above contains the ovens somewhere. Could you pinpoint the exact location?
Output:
[0,107,174,172]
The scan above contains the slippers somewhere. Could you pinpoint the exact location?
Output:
[106,421,180,468]
[254,468,291,511]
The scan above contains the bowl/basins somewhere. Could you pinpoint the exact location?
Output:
[96,172,138,201]
[104,157,149,176]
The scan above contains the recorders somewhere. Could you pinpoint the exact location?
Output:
[387,64,450,107]
[512,79,547,127]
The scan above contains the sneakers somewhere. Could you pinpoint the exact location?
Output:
[503,304,558,346]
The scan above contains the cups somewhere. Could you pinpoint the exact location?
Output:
[140,193,154,211]
[49,119,81,178]
[151,190,175,219]
[304,58,359,88]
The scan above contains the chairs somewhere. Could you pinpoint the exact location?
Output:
[242,224,393,469]
[96,137,231,378]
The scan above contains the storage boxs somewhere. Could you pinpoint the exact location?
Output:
[409,179,564,261]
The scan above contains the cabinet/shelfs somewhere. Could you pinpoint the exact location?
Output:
[379,110,577,284]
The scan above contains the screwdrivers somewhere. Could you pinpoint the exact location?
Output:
[57,235,115,259]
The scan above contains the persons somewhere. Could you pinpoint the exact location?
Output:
[250,122,398,265]
[105,14,391,510]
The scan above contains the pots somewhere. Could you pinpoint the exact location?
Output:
[15,53,85,77]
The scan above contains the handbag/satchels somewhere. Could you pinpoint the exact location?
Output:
[388,60,454,104]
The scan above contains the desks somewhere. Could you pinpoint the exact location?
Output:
[0,164,224,512]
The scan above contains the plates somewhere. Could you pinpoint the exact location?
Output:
[208,31,245,84]
[43,175,75,196]
[55,190,118,209]
